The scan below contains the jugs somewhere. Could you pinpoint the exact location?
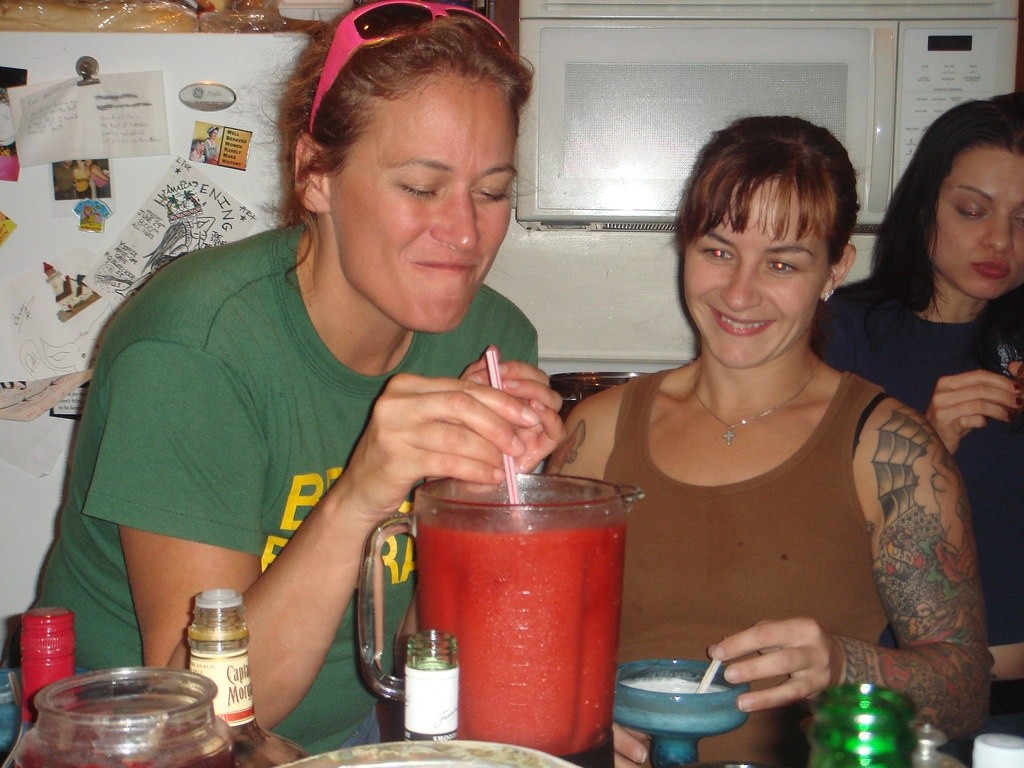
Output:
[355,472,645,759]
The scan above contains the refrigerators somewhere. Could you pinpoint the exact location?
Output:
[1,32,313,631]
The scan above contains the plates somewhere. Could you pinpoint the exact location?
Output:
[268,739,581,768]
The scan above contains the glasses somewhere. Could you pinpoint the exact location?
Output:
[309,0,517,135]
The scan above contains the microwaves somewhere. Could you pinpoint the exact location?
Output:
[517,1,1024,239]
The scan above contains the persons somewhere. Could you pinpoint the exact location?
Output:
[806,91,1024,768]
[543,116,1000,768]
[0,0,568,768]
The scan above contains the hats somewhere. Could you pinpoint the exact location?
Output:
[207,126,218,135]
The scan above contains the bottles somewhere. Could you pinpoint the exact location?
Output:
[187,587,310,768]
[11,665,236,768]
[804,683,914,768]
[2,608,76,768]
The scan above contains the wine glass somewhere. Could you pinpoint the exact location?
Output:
[614,657,750,768]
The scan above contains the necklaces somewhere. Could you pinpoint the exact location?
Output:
[693,357,818,446]
